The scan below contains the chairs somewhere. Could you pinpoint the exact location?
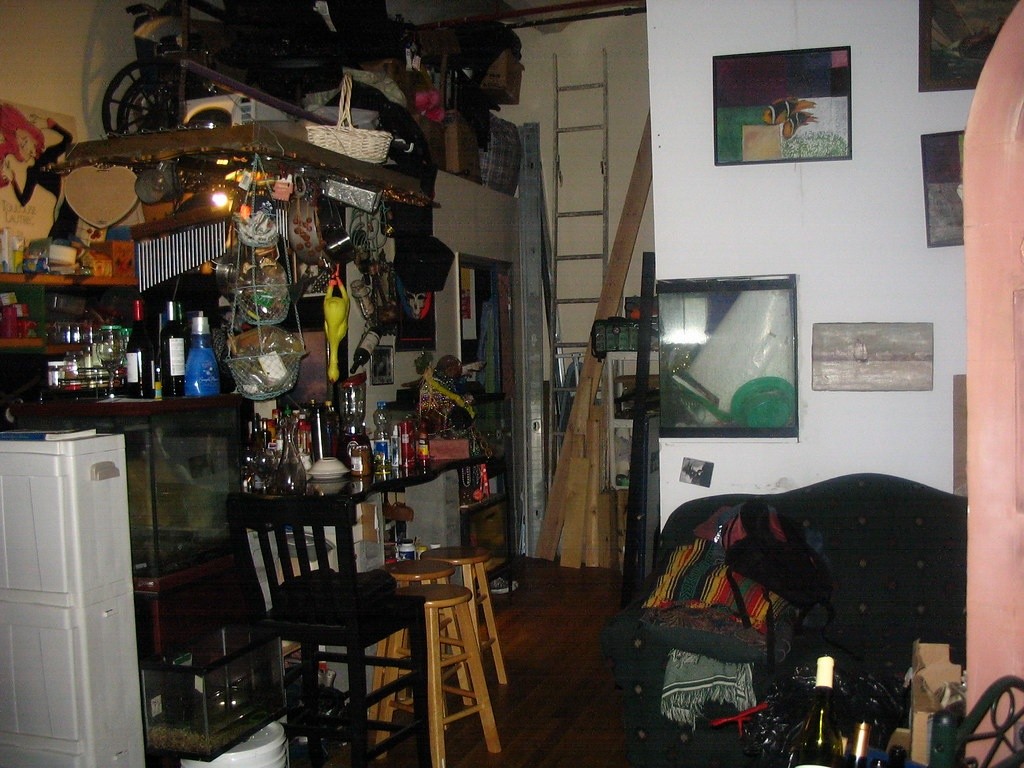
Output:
[225,490,432,768]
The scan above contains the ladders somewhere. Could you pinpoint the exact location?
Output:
[549,49,609,493]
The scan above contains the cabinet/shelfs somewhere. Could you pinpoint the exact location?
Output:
[0,271,139,355]
[606,350,659,489]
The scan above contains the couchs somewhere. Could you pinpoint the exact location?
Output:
[600,474,967,767]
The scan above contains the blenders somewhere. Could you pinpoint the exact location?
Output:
[336,374,373,476]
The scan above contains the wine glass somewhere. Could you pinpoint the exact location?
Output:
[96,330,125,399]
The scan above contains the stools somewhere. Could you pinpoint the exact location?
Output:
[375,585,502,767]
[420,548,509,685]
[368,560,479,731]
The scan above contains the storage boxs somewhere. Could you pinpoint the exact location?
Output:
[477,48,525,105]
[911,637,962,766]
[10,393,289,762]
[412,112,479,176]
[429,439,470,459]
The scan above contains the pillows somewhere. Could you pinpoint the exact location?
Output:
[638,601,794,664]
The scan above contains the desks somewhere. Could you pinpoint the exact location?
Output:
[247,456,487,748]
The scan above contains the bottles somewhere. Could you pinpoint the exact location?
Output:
[242,398,430,495]
[161,301,186,397]
[399,539,441,561]
[184,311,220,396]
[126,300,155,399]
[349,325,382,373]
[792,656,906,768]
[351,280,376,325]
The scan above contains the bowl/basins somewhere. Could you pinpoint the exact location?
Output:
[307,456,349,474]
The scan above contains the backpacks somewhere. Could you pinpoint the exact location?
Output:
[720,499,828,609]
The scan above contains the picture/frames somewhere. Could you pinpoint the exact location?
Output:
[281,326,334,407]
[920,130,965,249]
[395,275,436,352]
[918,0,1021,91]
[712,45,853,166]
[370,345,394,385]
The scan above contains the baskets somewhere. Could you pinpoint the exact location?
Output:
[305,70,394,162]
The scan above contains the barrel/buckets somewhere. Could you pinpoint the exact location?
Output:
[181,720,288,768]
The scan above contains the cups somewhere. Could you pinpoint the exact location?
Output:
[63,354,85,385]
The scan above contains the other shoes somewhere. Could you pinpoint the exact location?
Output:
[489,577,518,594]
